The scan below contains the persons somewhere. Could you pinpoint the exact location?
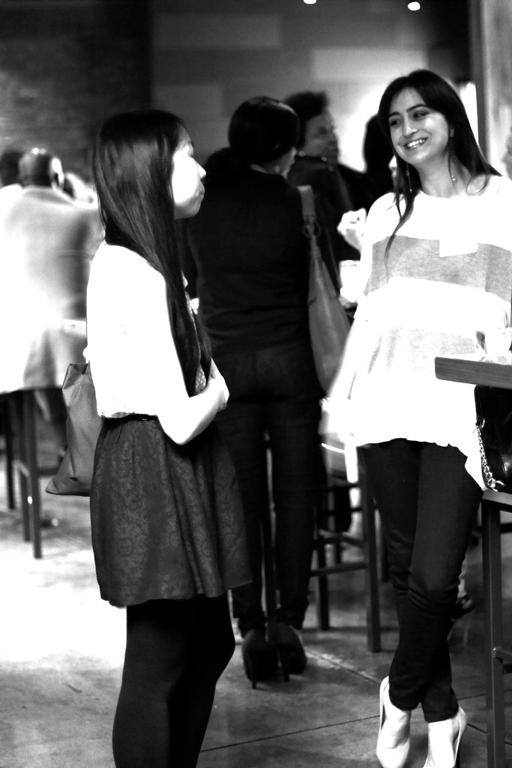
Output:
[174,94,335,691]
[290,93,397,314]
[321,69,512,768]
[0,138,104,463]
[84,111,247,768]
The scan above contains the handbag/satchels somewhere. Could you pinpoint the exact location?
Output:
[47,362,104,496]
[296,184,351,391]
[475,385,512,494]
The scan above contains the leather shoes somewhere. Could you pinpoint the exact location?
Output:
[376,676,411,768]
[423,704,467,768]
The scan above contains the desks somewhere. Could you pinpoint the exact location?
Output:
[435,350,512,768]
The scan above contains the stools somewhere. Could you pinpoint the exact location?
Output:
[261,407,382,653]
[18,384,60,560]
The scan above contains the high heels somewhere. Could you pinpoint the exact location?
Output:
[242,622,307,689]
